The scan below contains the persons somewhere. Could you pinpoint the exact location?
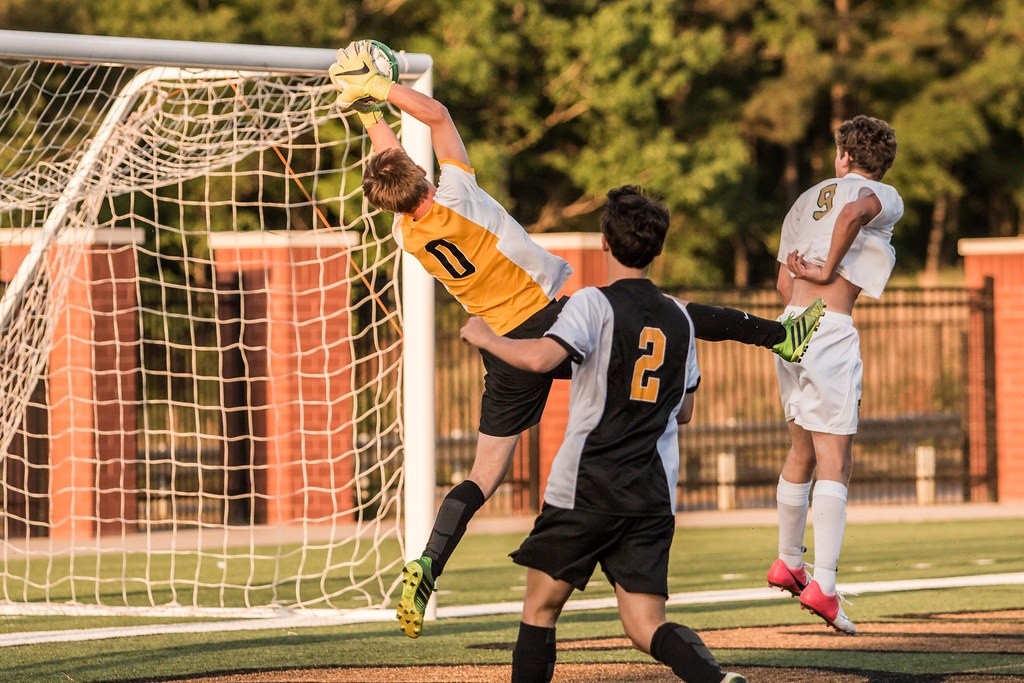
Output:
[459,186,748,683]
[767,117,904,633]
[330,41,831,639]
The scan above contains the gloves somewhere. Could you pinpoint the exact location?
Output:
[327,40,395,129]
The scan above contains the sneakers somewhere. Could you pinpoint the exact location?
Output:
[773,295,827,363]
[719,671,747,683]
[799,579,857,635]
[766,559,815,598]
[395,555,441,640]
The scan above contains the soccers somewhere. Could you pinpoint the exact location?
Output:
[337,39,399,106]
